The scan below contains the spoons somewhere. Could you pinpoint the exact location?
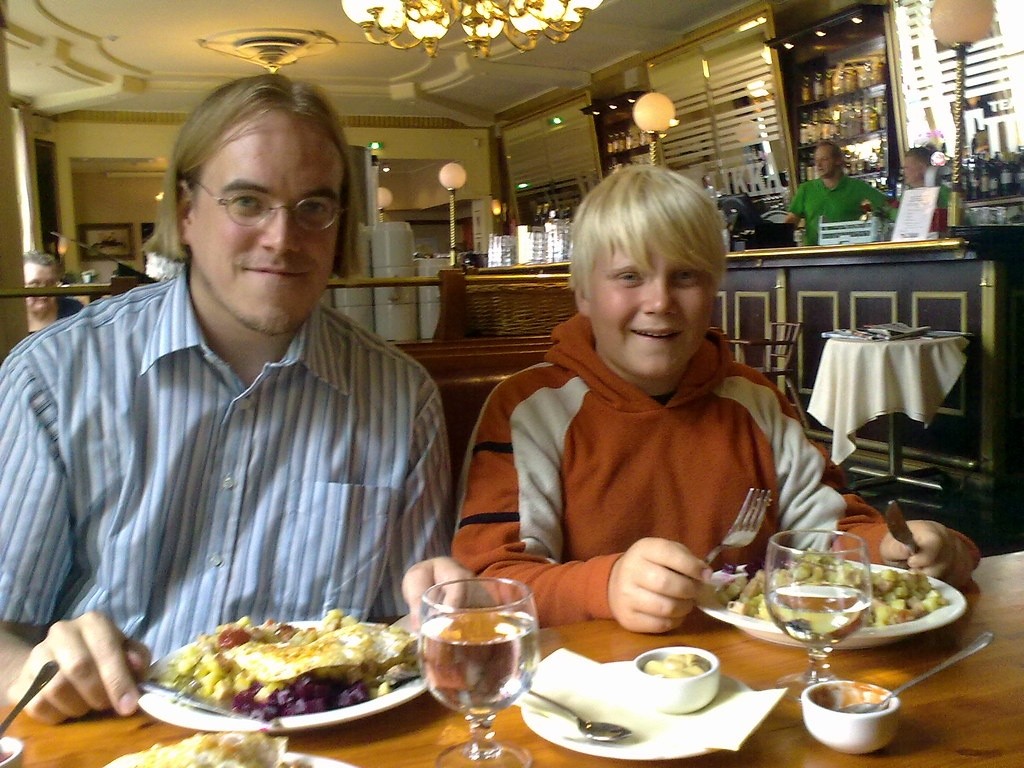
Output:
[519,691,632,741]
[838,632,994,714]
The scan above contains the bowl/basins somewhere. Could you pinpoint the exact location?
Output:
[632,646,721,715]
[801,680,904,755]
[0,735,23,768]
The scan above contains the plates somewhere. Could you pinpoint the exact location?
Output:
[100,748,359,768]
[520,661,754,760]
[137,619,429,734]
[693,559,968,650]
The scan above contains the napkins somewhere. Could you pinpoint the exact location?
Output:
[511,647,789,751]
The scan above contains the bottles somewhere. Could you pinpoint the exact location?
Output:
[797,62,1023,198]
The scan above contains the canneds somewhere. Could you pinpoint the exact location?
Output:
[800,161,821,182]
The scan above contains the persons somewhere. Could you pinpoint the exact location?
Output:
[451,163,979,636]
[21,250,85,335]
[0,73,496,726]
[885,141,974,226]
[783,139,899,245]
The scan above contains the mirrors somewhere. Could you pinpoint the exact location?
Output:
[378,158,464,257]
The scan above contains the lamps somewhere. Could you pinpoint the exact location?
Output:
[339,0,603,60]
[378,186,392,223]
[633,93,676,166]
[930,0,994,230]
[437,163,467,267]
[735,121,766,190]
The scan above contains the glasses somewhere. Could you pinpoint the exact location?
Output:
[187,177,345,233]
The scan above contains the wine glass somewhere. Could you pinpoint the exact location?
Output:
[417,575,541,768]
[763,530,873,702]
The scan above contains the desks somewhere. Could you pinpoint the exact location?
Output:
[805,336,969,503]
[0,550,1024,768]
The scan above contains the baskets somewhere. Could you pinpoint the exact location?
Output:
[460,284,576,337]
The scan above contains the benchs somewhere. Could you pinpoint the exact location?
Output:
[390,335,557,508]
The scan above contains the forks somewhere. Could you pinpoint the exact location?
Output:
[703,490,771,567]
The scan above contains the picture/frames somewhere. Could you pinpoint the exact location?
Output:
[79,221,136,262]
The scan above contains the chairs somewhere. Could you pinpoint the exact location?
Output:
[726,321,810,431]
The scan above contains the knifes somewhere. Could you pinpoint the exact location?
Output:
[885,500,918,557]
[133,676,248,719]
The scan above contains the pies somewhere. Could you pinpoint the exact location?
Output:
[224,622,420,685]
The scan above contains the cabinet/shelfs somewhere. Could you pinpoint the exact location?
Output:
[761,2,899,188]
[579,89,650,179]
